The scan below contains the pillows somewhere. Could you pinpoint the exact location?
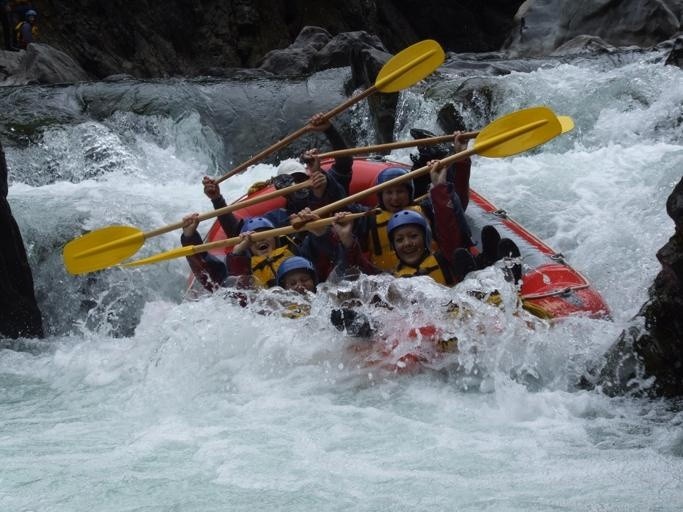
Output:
[387,209,433,255]
[276,158,307,179]
[241,217,278,244]
[276,257,316,289]
[376,168,415,203]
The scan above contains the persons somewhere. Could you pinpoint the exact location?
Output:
[179,112,520,335]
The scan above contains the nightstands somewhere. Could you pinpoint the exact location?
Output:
[212,39,446,185]
[311,114,575,160]
[289,106,561,230]
[64,177,314,275]
[119,207,384,268]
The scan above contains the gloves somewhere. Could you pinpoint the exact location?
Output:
[173,148,617,378]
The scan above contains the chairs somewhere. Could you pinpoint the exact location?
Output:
[409,127,447,160]
[480,224,503,268]
[332,308,378,339]
[452,247,479,281]
[496,238,521,282]
